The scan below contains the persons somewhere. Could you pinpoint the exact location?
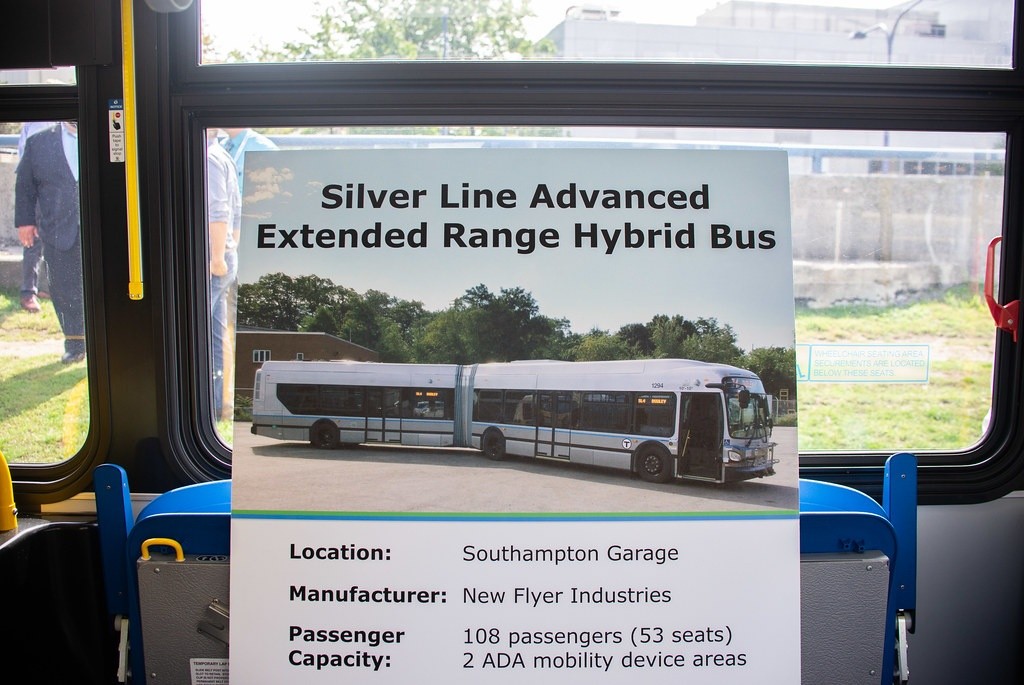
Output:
[220,126,286,222]
[15,118,60,313]
[204,126,243,423]
[14,120,87,364]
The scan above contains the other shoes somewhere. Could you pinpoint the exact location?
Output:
[22,294,40,313]
[38,290,52,299]
[61,350,86,364]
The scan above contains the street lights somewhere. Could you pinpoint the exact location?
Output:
[851,2,922,146]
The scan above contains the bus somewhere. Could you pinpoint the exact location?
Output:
[250,358,780,486]
